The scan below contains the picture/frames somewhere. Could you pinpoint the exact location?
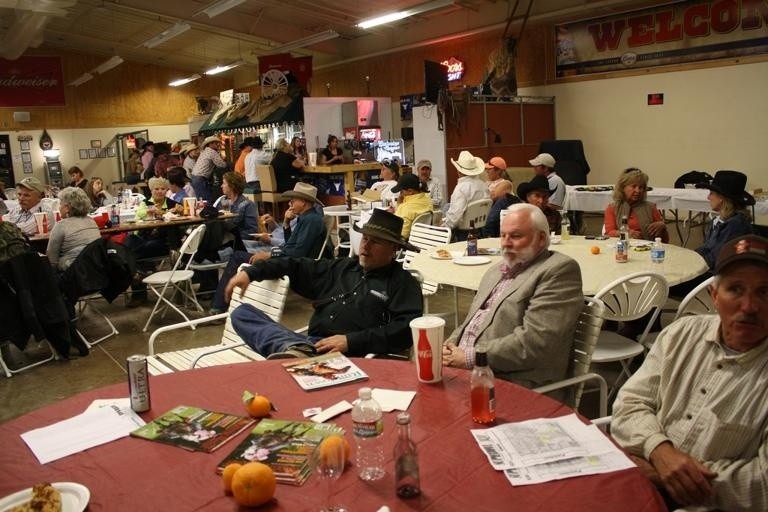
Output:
[20,141,33,174]
[79,139,116,159]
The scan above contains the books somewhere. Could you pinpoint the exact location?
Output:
[283,352,370,391]
[216,418,347,485]
[130,404,257,453]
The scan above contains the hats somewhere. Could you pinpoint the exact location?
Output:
[280,181,325,207]
[714,234,768,275]
[391,160,431,193]
[351,189,380,205]
[694,170,755,205]
[517,153,557,202]
[239,136,265,149]
[15,177,44,194]
[450,150,507,176]
[353,208,420,253]
[178,136,221,156]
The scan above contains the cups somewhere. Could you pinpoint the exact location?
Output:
[53,211,61,223]
[409,316,446,383]
[100,187,207,220]
[34,212,48,235]
[221,199,230,213]
[308,152,317,166]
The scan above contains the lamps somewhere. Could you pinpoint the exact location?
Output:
[67,1,249,88]
[168,1,456,88]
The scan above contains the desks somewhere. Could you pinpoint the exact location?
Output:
[300,163,382,205]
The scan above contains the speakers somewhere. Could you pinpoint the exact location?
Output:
[14,112,31,122]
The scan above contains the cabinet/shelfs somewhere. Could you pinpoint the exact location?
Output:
[412,103,556,204]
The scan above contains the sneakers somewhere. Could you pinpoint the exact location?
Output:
[126,298,146,308]
[189,311,225,326]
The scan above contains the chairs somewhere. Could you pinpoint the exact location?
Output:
[0,219,242,377]
[673,171,714,240]
[292,268,424,358]
[571,271,669,402]
[256,163,292,222]
[315,179,493,299]
[530,294,608,432]
[145,261,290,377]
[636,275,720,359]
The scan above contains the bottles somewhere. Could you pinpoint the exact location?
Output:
[619,215,631,250]
[392,411,422,498]
[56,213,61,221]
[417,328,435,381]
[467,220,477,255]
[350,387,387,480]
[651,237,665,277]
[34,215,40,234]
[560,209,572,240]
[42,214,47,233]
[346,190,351,210]
[470,343,497,425]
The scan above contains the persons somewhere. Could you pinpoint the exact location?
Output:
[224,210,425,359]
[210,136,343,315]
[370,151,566,242]
[125,136,258,308]
[603,167,756,361]
[610,234,767,512]
[1,167,113,344]
[442,204,582,399]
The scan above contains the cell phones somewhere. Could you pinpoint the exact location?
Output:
[597,236,609,239]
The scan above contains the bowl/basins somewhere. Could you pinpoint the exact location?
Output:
[548,235,562,244]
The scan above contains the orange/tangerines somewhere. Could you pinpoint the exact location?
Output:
[221,461,277,505]
[245,391,272,417]
[590,247,600,254]
[322,436,352,467]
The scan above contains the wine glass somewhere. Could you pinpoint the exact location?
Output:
[305,430,350,511]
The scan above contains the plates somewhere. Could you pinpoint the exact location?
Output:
[431,251,465,260]
[454,256,490,265]
[475,247,500,255]
[1,482,90,511]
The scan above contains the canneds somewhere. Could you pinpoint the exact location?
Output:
[125,354,150,413]
[615,239,628,263]
